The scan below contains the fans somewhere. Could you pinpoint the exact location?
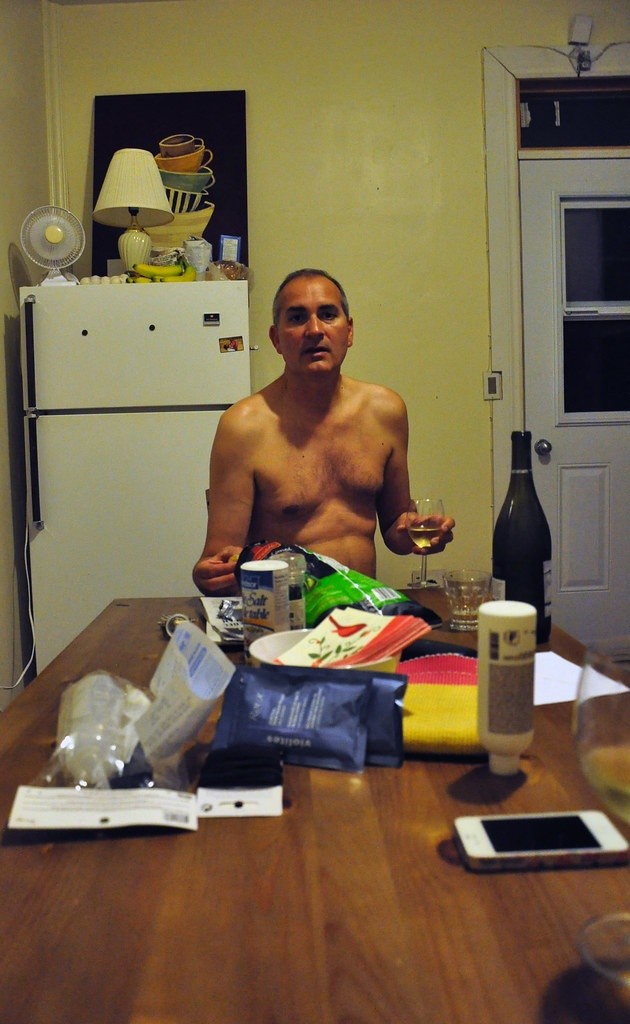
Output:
[20,205,85,286]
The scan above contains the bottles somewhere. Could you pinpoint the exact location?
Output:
[269,553,307,630]
[492,431,551,646]
[476,600,538,777]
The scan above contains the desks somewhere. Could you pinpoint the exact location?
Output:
[0,587,630,1024]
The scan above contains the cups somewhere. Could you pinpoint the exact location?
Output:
[66,676,123,782]
[142,133,215,250]
[442,570,492,632]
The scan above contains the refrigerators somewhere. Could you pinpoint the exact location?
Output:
[248,628,402,674]
[17,278,252,674]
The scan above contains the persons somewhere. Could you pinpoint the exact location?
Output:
[192,269,455,597]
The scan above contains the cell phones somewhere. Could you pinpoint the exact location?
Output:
[453,810,630,872]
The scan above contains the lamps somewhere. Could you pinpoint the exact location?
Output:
[91,148,174,271]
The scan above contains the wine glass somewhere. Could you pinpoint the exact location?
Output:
[406,498,445,590]
[573,636,630,985]
